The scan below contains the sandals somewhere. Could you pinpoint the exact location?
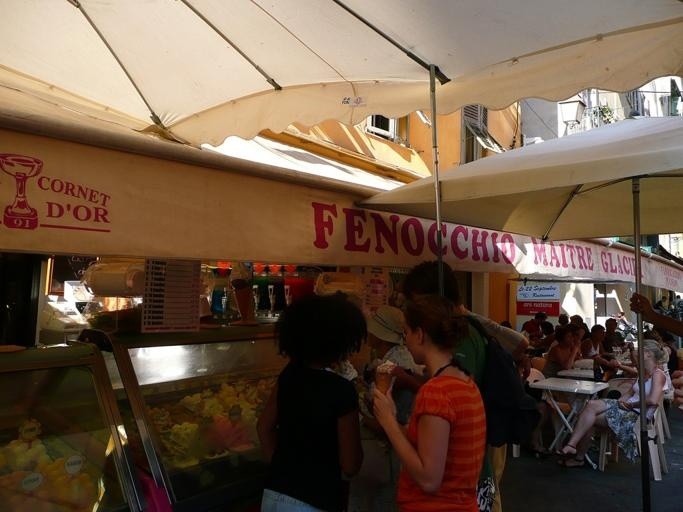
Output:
[557,459,585,467]
[555,444,578,457]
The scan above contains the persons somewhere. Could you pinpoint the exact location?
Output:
[356,303,425,511]
[497,293,681,469]
[369,292,486,512]
[255,291,364,512]
[389,260,531,512]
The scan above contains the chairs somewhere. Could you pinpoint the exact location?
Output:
[522,340,671,482]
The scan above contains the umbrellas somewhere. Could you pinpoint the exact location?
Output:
[352,110,682,512]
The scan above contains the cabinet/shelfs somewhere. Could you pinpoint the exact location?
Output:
[79,325,290,511]
[0,343,145,511]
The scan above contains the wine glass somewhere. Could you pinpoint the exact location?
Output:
[0,152,43,216]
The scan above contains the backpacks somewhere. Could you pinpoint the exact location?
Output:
[481,340,542,437]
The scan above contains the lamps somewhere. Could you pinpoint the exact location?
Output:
[557,92,586,130]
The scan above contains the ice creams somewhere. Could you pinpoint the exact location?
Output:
[338,359,359,387]
[375,360,398,393]
